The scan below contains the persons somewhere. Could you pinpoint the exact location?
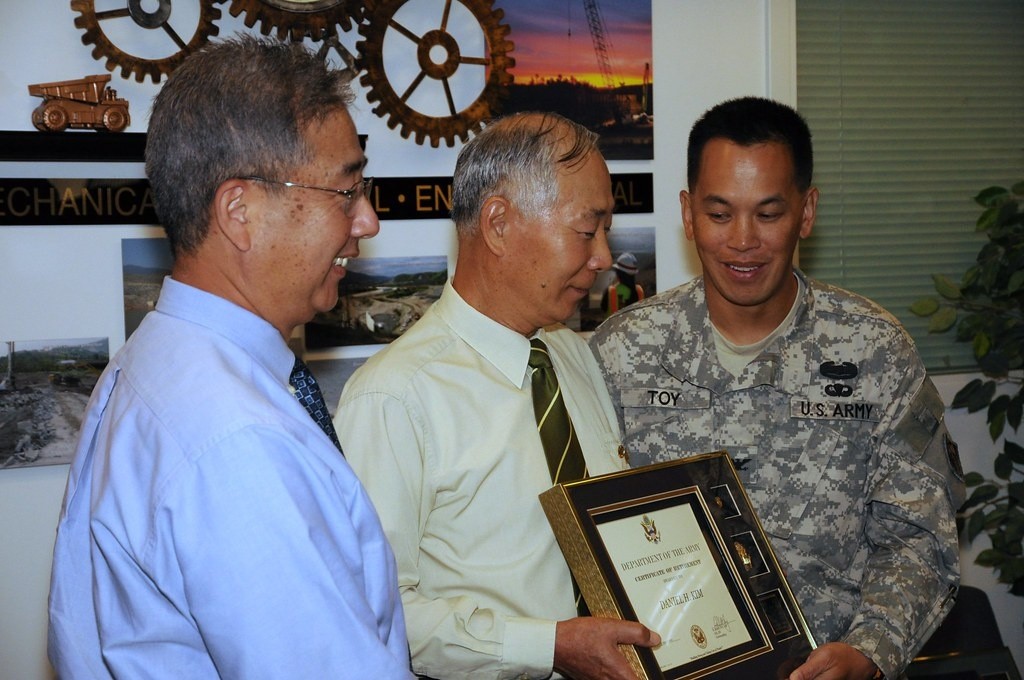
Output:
[586,96,967,680]
[331,108,662,680]
[47,31,422,680]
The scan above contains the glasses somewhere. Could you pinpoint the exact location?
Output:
[206,175,374,218]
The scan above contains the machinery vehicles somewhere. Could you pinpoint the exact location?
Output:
[28,74,130,132]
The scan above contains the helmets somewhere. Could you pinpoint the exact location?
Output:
[612,252,639,275]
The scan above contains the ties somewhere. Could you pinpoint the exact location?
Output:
[528,338,592,617]
[289,355,414,673]
